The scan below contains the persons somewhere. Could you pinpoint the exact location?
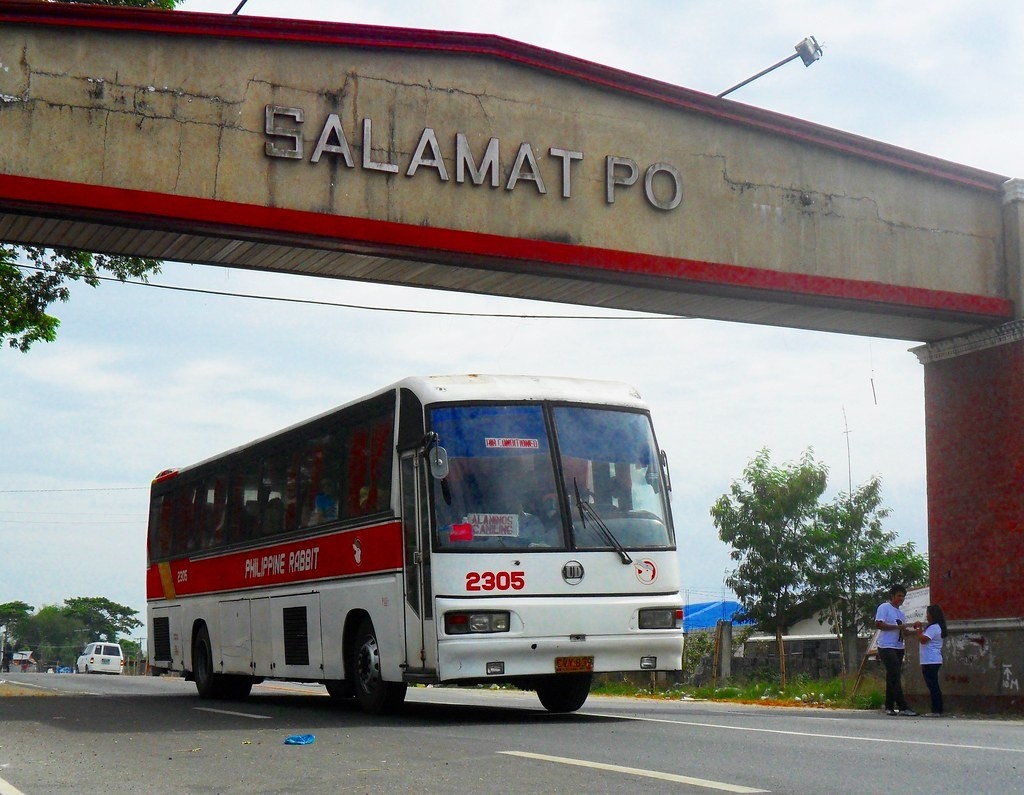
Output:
[314,478,337,525]
[2,642,13,672]
[875,585,919,716]
[912,605,948,717]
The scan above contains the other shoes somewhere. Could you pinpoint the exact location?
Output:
[899,708,918,716]
[887,710,897,715]
[925,713,940,717]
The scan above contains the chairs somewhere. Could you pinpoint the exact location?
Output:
[192,478,390,551]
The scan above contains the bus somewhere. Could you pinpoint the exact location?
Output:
[146,373,687,713]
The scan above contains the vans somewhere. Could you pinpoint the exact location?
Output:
[75,642,124,675]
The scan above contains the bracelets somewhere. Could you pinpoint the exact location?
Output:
[917,628,920,630]
